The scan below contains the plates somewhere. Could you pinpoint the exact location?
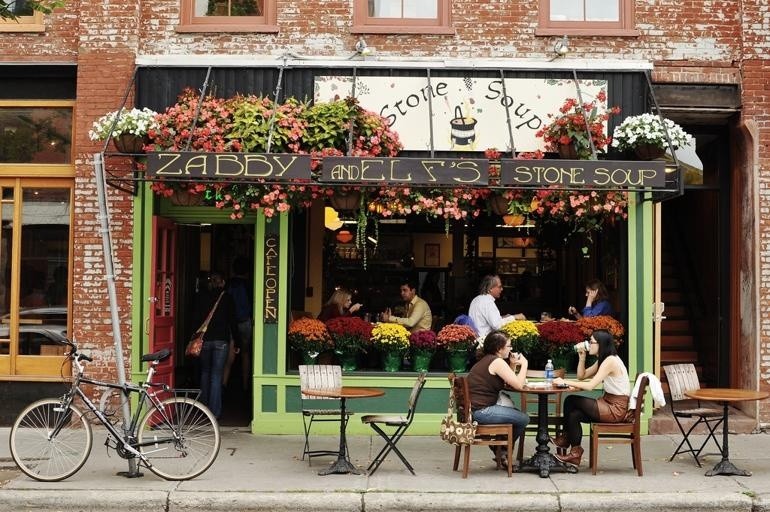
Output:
[556,383,573,389]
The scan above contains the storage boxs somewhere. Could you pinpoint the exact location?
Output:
[40,344,67,355]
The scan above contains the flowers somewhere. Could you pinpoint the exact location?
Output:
[537,320,587,374]
[287,316,335,365]
[325,316,374,371]
[407,330,437,371]
[576,314,626,366]
[497,320,540,374]
[369,321,411,372]
[435,324,479,374]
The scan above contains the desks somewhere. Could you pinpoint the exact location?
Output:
[300,386,385,476]
[683,387,770,477]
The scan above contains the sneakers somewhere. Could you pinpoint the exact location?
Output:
[489,438,513,471]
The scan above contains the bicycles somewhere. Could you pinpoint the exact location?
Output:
[6,330,224,481]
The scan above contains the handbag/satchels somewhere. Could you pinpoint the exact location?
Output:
[185,327,206,357]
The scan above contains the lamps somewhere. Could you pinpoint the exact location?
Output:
[547,34,570,62]
[347,38,370,61]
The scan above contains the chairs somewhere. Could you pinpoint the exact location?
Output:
[360,368,427,477]
[662,363,725,469]
[298,363,353,469]
[589,371,649,477]
[447,371,514,479]
[516,368,564,462]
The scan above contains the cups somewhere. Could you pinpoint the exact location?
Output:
[540,312,551,322]
[573,340,590,354]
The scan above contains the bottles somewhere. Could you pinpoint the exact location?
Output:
[544,359,554,387]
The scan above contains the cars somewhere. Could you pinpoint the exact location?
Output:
[1,305,67,325]
[0,324,67,353]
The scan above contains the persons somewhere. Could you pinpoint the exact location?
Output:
[220,254,250,395]
[568,279,612,320]
[466,330,531,470]
[468,272,526,337]
[380,281,433,334]
[548,328,631,467]
[185,270,242,419]
[316,287,364,323]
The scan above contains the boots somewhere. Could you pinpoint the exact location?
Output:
[548,431,569,448]
[555,445,584,467]
